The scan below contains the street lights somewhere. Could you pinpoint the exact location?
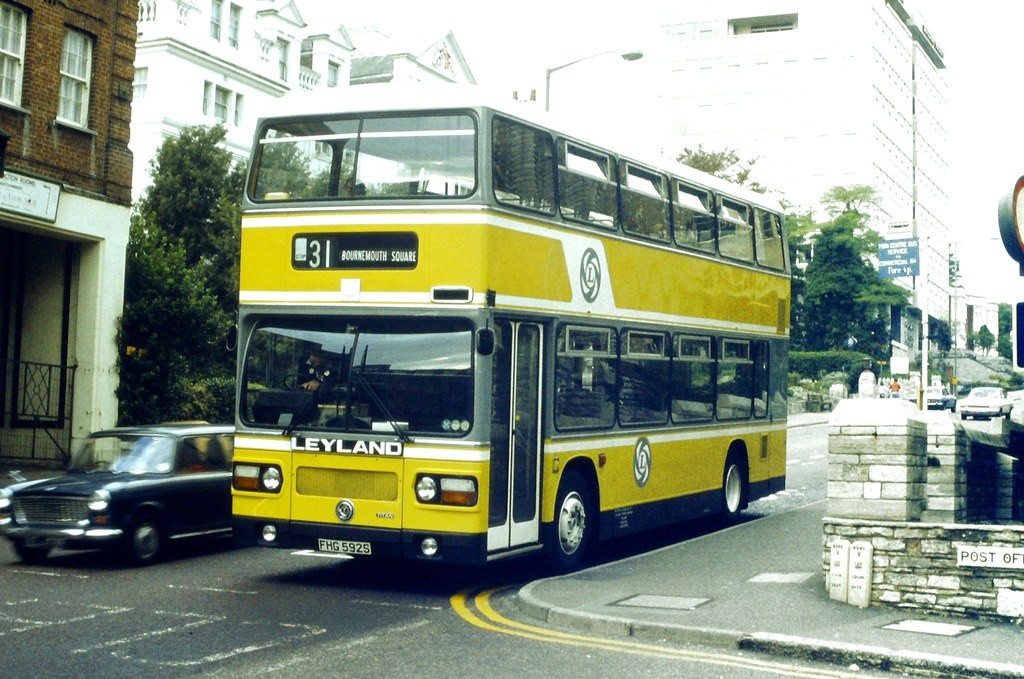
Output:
[544,44,645,113]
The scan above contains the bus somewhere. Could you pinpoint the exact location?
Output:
[229,103,795,579]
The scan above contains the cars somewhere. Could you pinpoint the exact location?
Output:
[878,383,959,414]
[0,419,252,568]
[955,385,1013,422]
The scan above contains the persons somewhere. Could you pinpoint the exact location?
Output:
[299,346,339,403]
[889,378,901,398]
[345,177,366,196]
